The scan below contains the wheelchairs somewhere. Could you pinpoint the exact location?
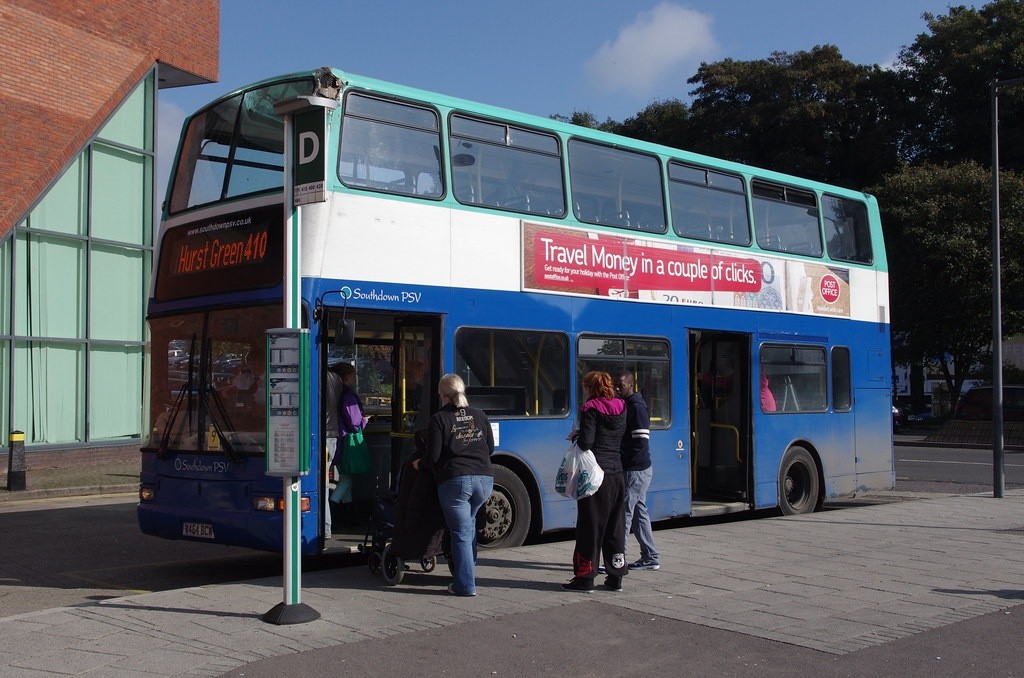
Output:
[354,460,451,584]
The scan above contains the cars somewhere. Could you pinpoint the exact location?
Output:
[891,392,934,432]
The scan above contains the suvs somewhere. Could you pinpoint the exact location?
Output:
[952,385,1024,422]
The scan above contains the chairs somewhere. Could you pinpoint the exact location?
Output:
[389,176,815,258]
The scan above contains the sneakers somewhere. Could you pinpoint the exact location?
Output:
[597,565,606,574]
[628,559,660,569]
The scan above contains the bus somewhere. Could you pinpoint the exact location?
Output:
[135,67,896,562]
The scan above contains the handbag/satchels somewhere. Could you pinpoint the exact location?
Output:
[341,426,373,476]
[555,439,604,499]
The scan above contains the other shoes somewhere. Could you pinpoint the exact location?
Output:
[344,503,357,513]
[603,575,623,592]
[560,577,595,593]
[329,501,344,516]
[447,582,477,596]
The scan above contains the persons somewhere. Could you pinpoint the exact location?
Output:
[598,371,660,573]
[328,363,370,531]
[391,361,424,431]
[718,367,777,470]
[413,374,494,594]
[561,371,628,592]
